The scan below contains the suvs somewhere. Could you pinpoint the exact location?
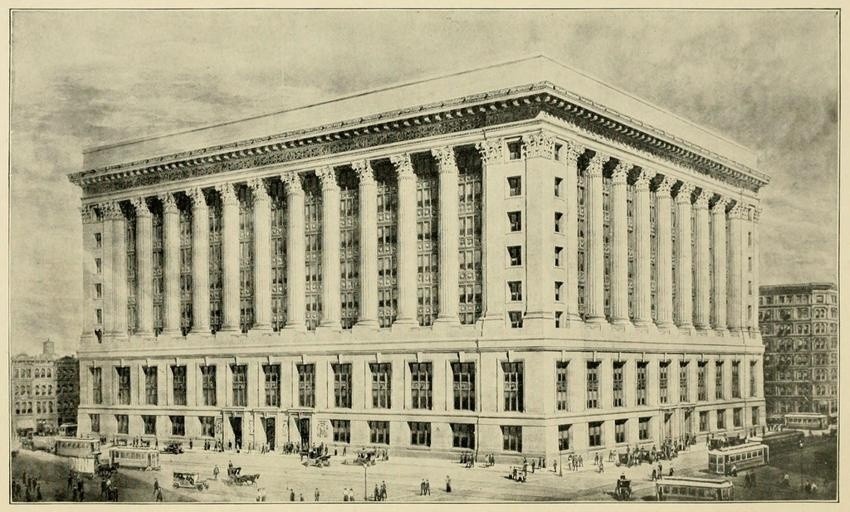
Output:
[172,470,209,491]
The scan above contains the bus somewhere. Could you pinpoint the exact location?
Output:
[762,430,806,452]
[59,422,77,437]
[109,445,160,471]
[55,438,102,459]
[783,412,828,429]
[655,476,735,501]
[708,441,771,475]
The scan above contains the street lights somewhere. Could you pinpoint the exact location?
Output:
[559,435,564,476]
[798,438,805,493]
[361,460,369,500]
[38,419,46,436]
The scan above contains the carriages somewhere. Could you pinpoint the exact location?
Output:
[226,467,260,486]
[302,453,331,467]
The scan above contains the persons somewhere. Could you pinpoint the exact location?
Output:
[566,449,616,474]
[619,461,674,482]
[420,475,452,496]
[625,431,697,467]
[729,462,757,489]
[11,423,120,502]
[781,471,818,494]
[507,456,557,483]
[706,422,785,447]
[284,438,391,465]
[461,450,495,468]
[132,435,269,502]
[289,480,388,502]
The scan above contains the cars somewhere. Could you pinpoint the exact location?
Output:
[163,443,183,453]
